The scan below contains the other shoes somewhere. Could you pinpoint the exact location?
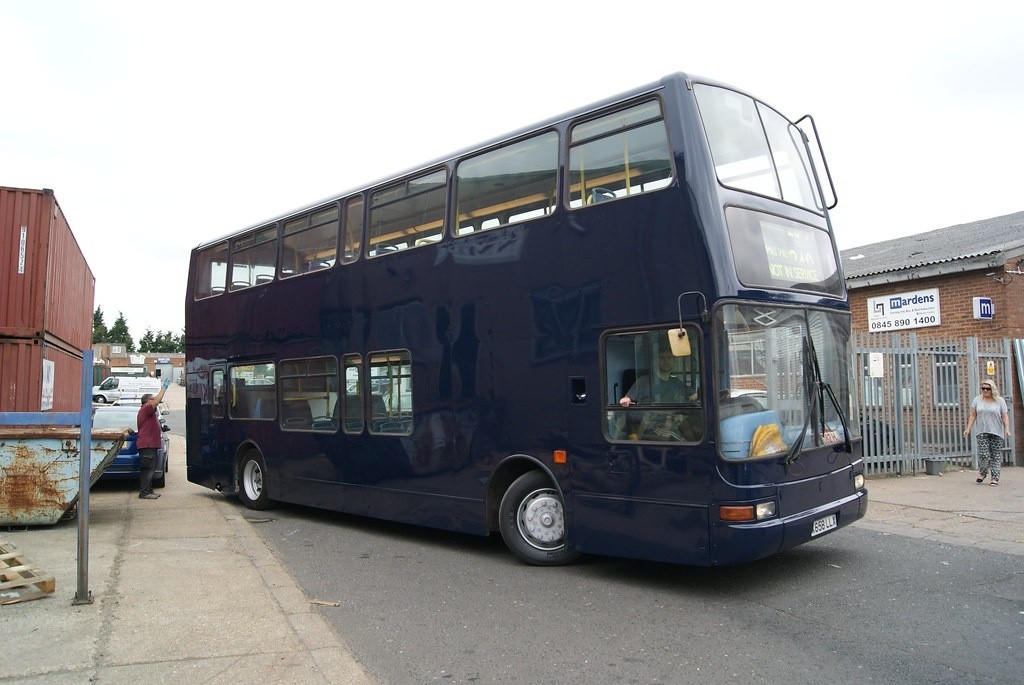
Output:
[990,481,998,486]
[153,492,161,496]
[143,493,157,499]
[976,475,987,484]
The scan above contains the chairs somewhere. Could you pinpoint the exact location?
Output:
[206,239,434,297]
[589,186,617,203]
[277,379,412,432]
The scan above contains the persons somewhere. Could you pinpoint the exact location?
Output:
[964,380,1011,486]
[619,347,700,441]
[137,377,171,500]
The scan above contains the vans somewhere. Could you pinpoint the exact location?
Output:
[91,376,162,405]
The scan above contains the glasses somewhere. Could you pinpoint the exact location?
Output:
[982,387,991,391]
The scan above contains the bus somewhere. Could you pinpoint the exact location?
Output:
[184,69,871,568]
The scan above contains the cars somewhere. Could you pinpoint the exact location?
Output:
[90,405,172,488]
[856,409,899,469]
[109,393,171,427]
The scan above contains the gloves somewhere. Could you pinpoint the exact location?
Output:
[162,378,170,388]
